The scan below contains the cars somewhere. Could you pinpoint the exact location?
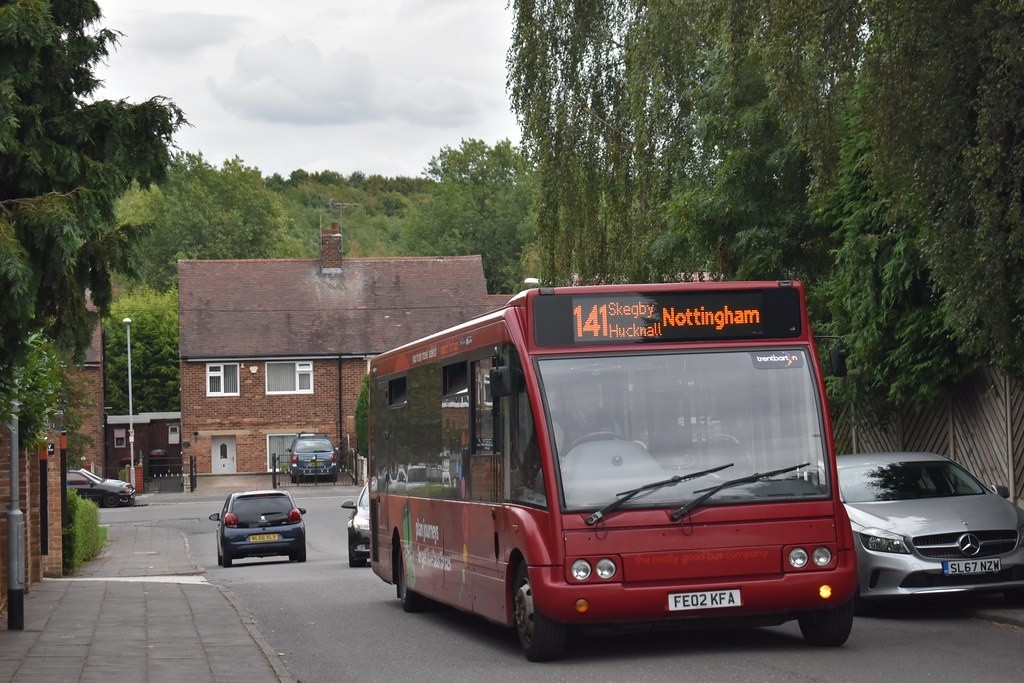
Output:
[209,489,307,569]
[818,451,1024,600]
[66,468,135,508]
[340,481,370,567]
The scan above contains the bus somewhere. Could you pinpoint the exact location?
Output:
[365,275,857,661]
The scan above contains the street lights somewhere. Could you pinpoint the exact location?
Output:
[122,316,136,489]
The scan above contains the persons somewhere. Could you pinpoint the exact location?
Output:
[523,382,648,459]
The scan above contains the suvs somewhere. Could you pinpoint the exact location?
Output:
[286,431,338,484]
